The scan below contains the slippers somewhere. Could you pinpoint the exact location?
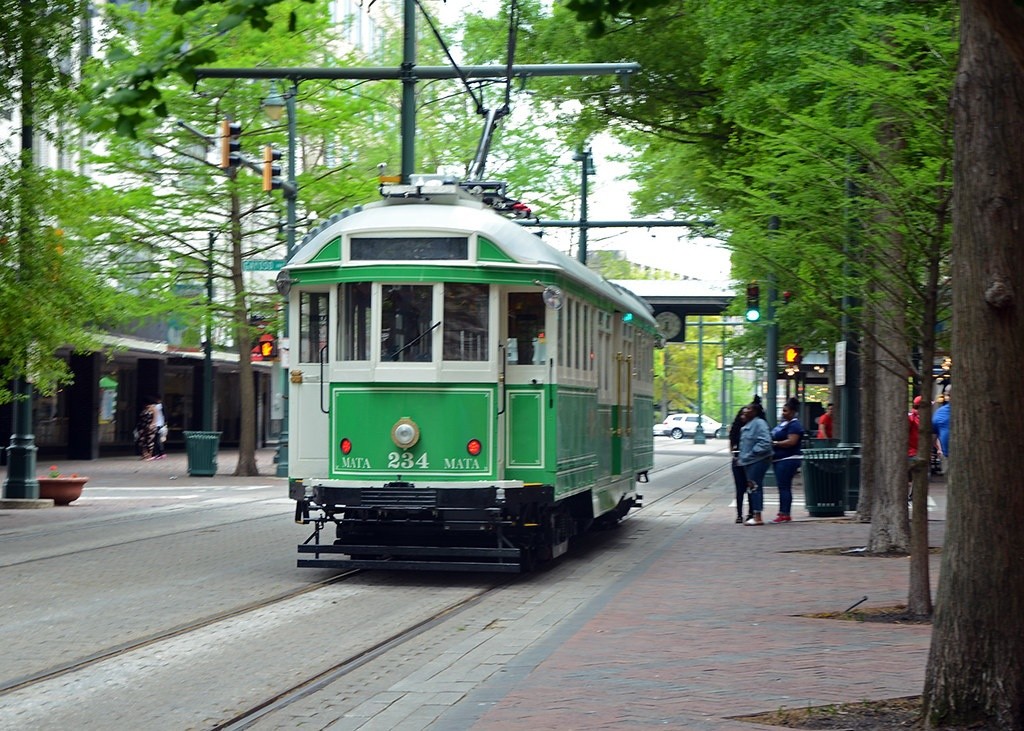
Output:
[744,518,764,525]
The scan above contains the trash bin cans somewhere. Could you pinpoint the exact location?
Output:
[800,449,853,517]
[183,430,222,478]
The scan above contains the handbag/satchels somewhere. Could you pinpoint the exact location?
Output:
[158,425,169,435]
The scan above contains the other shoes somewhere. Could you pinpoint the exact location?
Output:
[140,453,165,461]
[736,517,743,523]
[746,515,753,521]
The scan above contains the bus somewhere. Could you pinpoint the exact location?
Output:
[275,80,659,574]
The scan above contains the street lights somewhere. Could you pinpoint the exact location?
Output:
[572,146,595,267]
[260,78,296,478]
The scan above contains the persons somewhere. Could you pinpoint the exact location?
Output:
[931,384,952,488]
[738,403,774,525]
[908,396,943,484]
[137,397,167,461]
[728,405,753,523]
[817,402,834,438]
[768,398,803,523]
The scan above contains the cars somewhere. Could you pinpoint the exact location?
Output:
[653,424,666,435]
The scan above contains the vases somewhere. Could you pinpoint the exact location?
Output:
[37,476,90,506]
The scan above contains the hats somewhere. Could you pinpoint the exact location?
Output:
[913,396,922,403]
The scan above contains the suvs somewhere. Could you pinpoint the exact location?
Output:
[663,415,729,440]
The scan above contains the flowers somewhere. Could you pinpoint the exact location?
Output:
[48,465,77,478]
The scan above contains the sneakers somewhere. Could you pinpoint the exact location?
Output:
[776,513,782,517]
[769,515,791,524]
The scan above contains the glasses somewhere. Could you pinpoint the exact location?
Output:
[912,404,921,409]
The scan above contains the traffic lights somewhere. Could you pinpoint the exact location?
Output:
[259,340,277,359]
[262,148,282,191]
[784,345,804,365]
[746,283,760,322]
[221,120,241,168]
